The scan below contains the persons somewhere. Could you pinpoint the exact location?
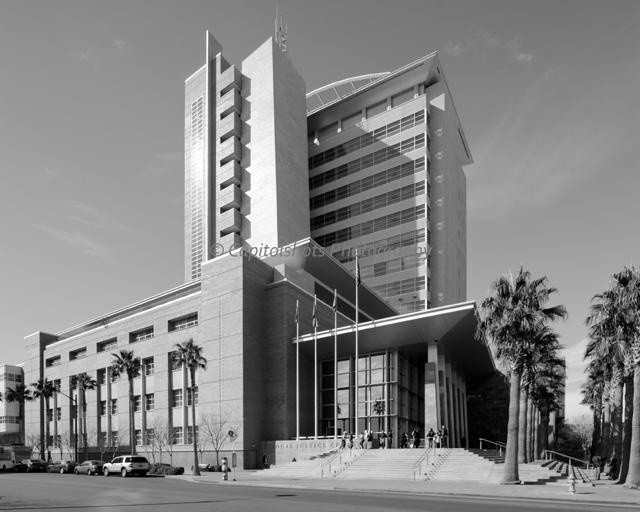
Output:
[262,454,268,468]
[593,456,603,480]
[340,426,448,449]
[608,455,618,479]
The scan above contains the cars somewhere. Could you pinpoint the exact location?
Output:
[14,457,49,472]
[46,459,77,474]
[74,460,106,475]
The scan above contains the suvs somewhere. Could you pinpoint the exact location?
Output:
[102,454,152,478]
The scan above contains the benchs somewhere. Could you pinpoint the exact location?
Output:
[191,464,210,473]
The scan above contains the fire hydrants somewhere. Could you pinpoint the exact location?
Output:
[567,472,580,495]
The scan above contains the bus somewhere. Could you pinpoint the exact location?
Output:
[1,443,33,473]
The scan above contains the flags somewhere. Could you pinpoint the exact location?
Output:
[295,302,299,323]
[312,297,318,327]
[356,255,360,285]
[333,292,337,312]
